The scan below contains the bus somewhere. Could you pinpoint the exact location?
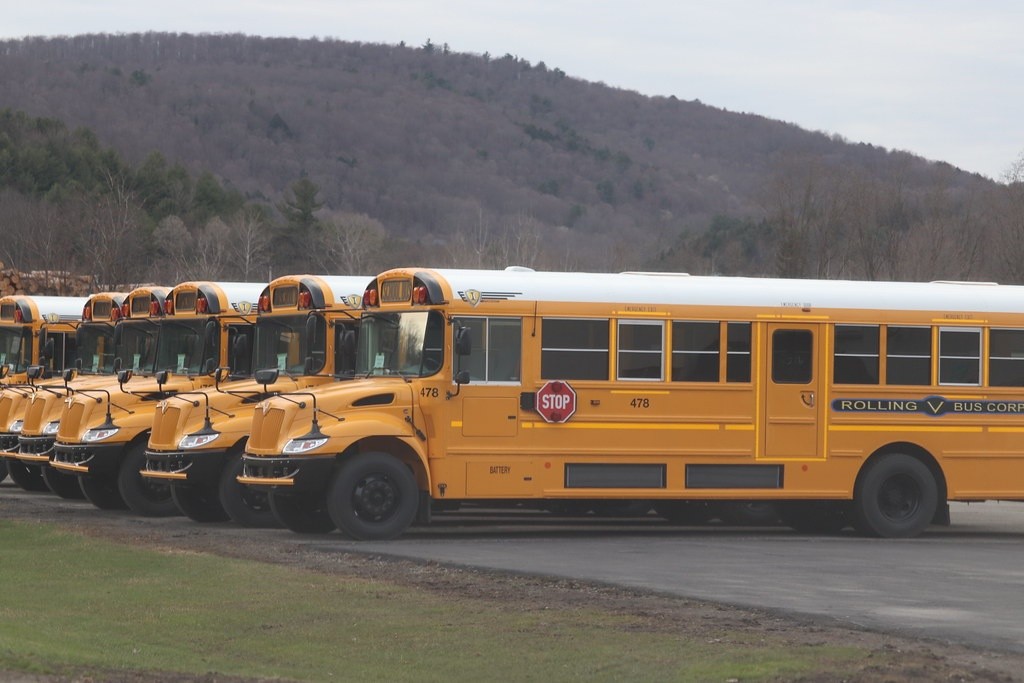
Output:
[215,266,1024,540]
[118,275,524,528]
[0,282,302,518]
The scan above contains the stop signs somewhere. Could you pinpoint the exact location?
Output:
[536,381,577,424]
